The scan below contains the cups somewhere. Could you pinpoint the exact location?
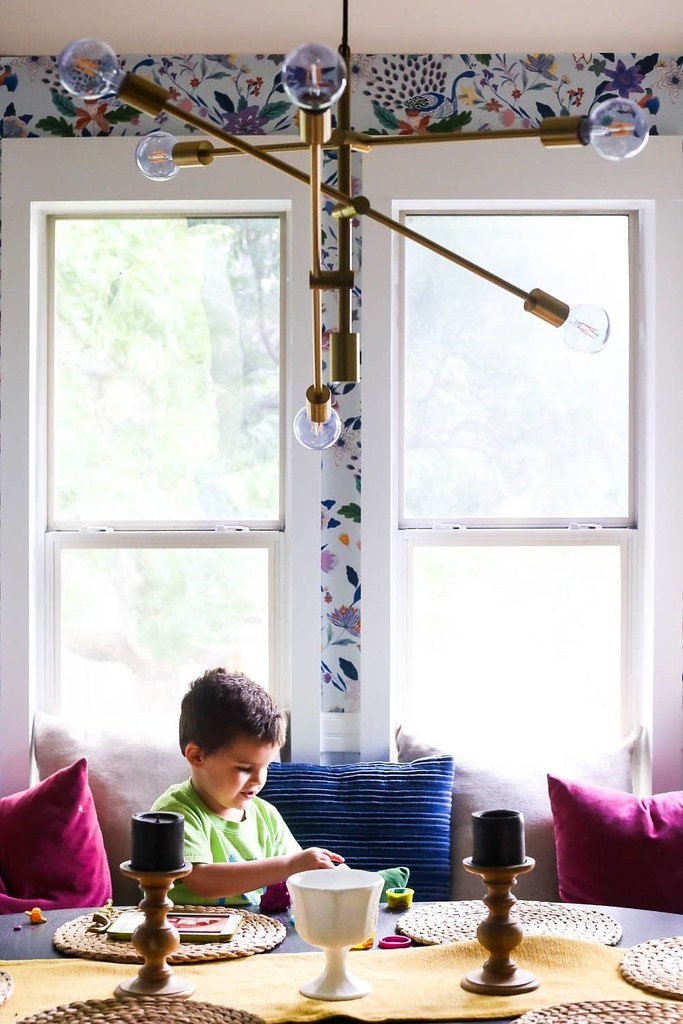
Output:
[385,888,414,909]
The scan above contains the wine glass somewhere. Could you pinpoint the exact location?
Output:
[286,868,386,1001]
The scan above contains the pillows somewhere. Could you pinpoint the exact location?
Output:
[260,754,456,903]
[32,708,195,907]
[389,725,647,903]
[545,774,683,916]
[0,758,112,915]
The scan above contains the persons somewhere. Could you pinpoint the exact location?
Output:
[149,667,351,904]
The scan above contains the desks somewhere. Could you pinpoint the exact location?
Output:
[0,900,683,1024]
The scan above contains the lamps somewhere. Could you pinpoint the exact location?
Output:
[57,0,651,451]
